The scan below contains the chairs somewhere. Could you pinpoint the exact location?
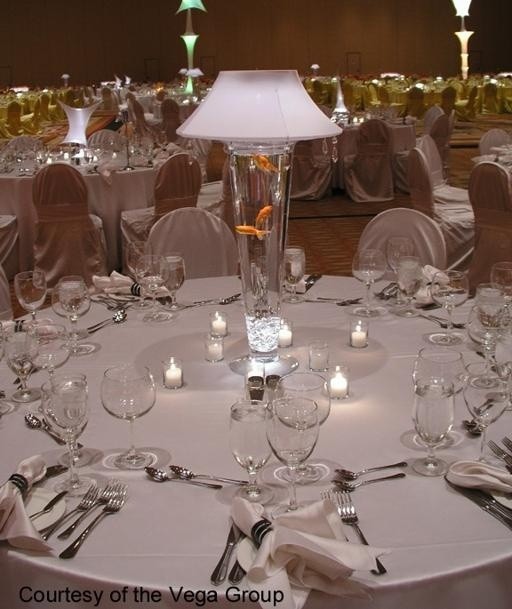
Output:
[0,75,512,321]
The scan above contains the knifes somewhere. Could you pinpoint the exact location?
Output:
[444,475,511,536]
[210,523,242,583]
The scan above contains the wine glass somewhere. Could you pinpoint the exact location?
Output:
[15,143,104,176]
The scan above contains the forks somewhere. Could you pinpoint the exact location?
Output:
[319,490,384,577]
[39,483,129,561]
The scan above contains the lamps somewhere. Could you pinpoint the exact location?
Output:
[311,64,320,74]
[176,69,344,381]
[452,0,474,79]
[176,0,208,94]
